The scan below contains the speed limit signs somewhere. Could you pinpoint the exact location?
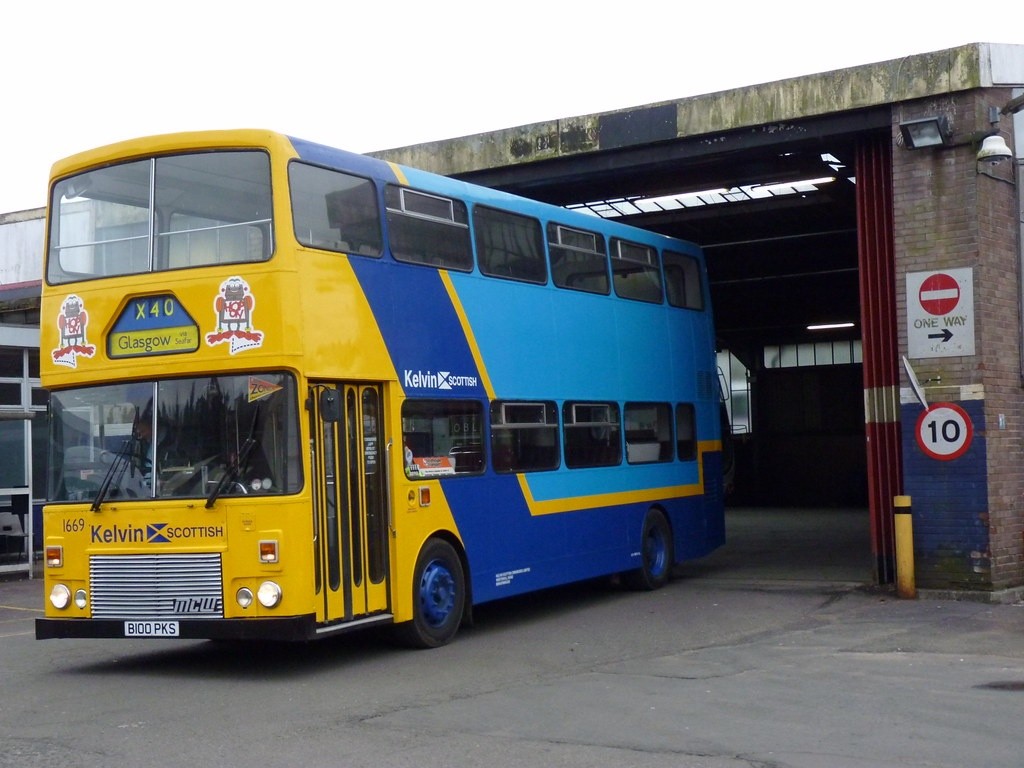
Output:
[915,401,974,461]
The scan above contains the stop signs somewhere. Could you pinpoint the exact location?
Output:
[918,273,963,316]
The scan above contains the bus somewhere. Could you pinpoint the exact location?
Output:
[34,126,728,652]
